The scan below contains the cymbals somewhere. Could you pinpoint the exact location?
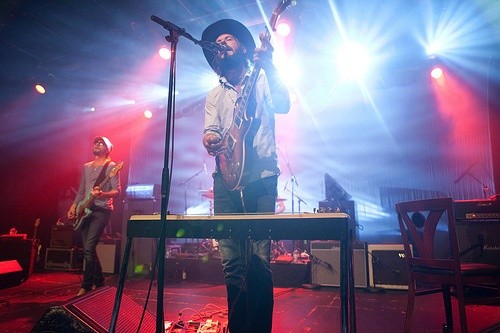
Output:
[275,198,287,202]
[202,191,215,199]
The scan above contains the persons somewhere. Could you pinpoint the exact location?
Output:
[68,137,121,295]
[201,18,290,333]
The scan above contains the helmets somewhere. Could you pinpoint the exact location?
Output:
[201,19,255,75]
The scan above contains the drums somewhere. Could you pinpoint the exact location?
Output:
[204,238,219,248]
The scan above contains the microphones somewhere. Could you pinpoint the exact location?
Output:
[205,41,227,54]
[454,163,476,184]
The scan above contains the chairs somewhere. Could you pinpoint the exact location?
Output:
[395,198,500,333]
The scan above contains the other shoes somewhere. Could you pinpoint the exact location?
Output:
[77,288,86,296]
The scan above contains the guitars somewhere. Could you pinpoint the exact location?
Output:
[215,0,298,192]
[69,160,126,232]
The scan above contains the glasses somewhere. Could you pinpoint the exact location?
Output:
[93,141,104,146]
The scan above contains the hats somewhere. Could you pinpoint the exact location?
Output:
[101,137,114,153]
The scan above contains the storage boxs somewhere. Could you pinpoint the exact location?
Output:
[367,244,414,291]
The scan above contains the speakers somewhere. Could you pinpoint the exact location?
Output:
[122,198,161,275]
[455,218,500,276]
[31,285,156,333]
[50,225,75,248]
[310,241,368,287]
[83,239,122,275]
[0,260,23,289]
[368,244,416,289]
